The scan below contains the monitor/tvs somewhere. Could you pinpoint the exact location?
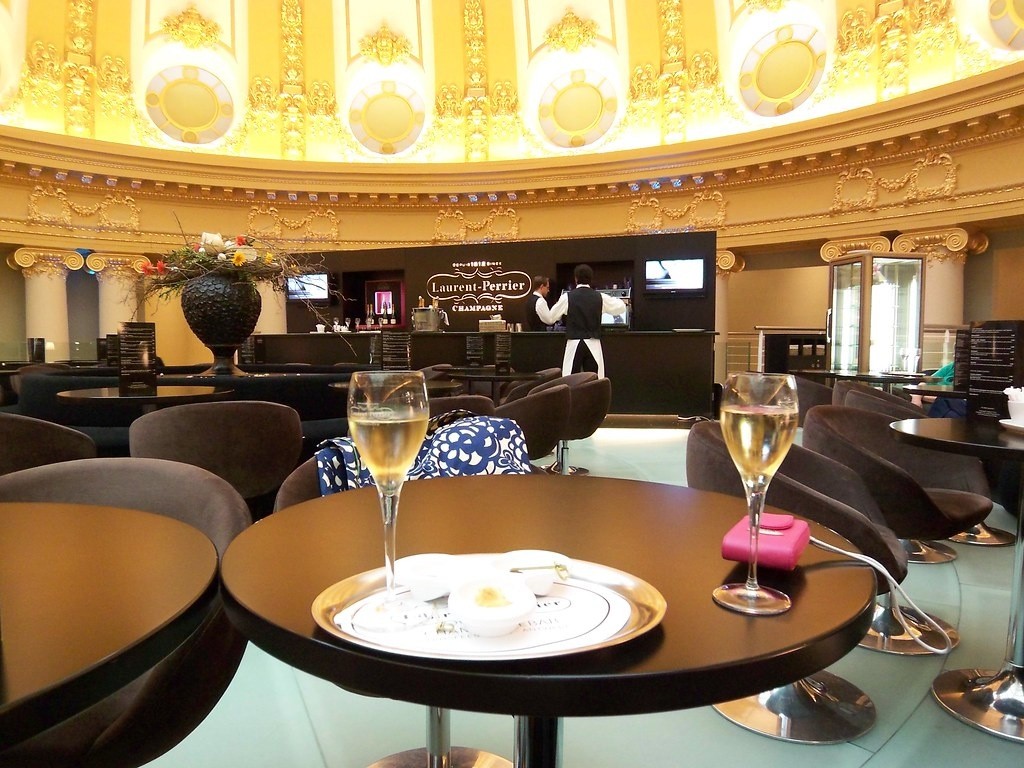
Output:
[644,257,707,298]
[287,272,329,301]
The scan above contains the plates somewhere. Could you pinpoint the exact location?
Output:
[309,331,332,334]
[999,419,1024,434]
[309,552,669,663]
[333,330,352,333]
[359,330,381,334]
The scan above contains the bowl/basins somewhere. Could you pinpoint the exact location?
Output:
[447,573,538,637]
[494,549,572,596]
[394,551,467,602]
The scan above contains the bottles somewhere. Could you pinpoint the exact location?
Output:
[367,302,397,325]
[418,294,439,307]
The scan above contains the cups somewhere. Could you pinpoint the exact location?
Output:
[45,341,55,364]
[507,324,514,332]
[333,325,341,331]
[1007,401,1024,425]
[315,323,326,332]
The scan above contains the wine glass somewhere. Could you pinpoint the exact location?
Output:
[333,316,339,331]
[345,318,351,331]
[346,369,440,635]
[355,318,360,334]
[709,372,800,616]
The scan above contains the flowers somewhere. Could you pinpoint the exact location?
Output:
[111,231,355,357]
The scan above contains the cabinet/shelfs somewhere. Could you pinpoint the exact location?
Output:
[764,334,826,374]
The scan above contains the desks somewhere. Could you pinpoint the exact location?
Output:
[328,381,465,393]
[788,369,842,389]
[835,374,921,393]
[0,500,218,750]
[902,385,968,400]
[446,371,544,407]
[224,470,877,768]
[54,385,237,416]
[886,413,1024,747]
[880,372,926,377]
[431,365,495,396]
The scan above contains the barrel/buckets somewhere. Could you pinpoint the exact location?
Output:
[412,307,449,332]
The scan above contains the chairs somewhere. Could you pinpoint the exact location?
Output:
[1,361,1024,768]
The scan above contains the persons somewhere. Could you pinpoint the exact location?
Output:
[910,361,954,408]
[527,265,626,380]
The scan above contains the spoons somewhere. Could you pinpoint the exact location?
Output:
[507,558,569,584]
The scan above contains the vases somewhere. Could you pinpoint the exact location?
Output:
[180,272,262,379]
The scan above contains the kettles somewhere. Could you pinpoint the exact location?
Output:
[515,322,522,332]
